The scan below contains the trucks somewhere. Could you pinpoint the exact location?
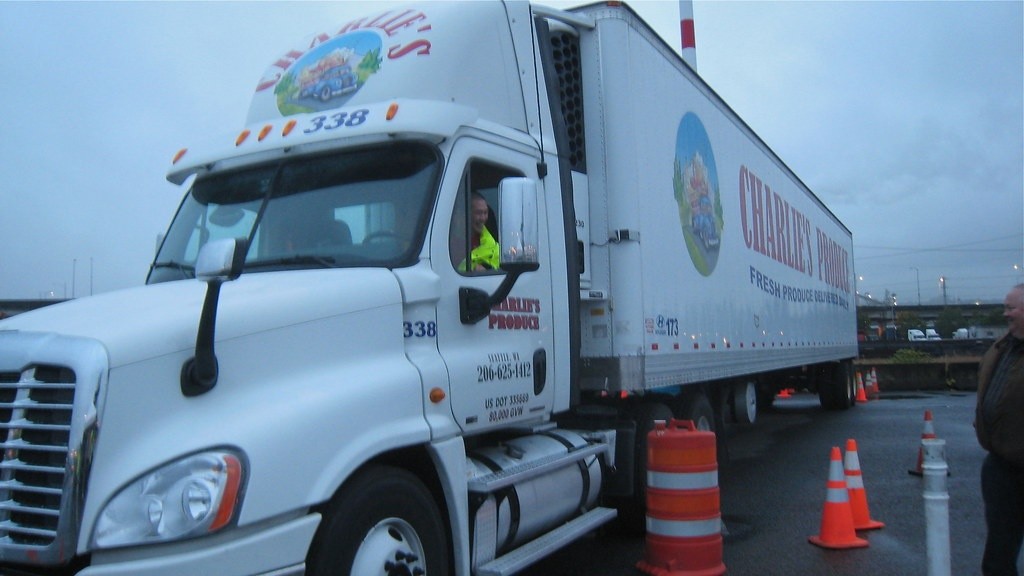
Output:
[951,325,1009,342]
[907,328,942,341]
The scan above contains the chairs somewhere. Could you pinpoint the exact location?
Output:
[403,182,499,271]
[259,174,355,257]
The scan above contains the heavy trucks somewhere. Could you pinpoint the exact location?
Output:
[2,0,864,576]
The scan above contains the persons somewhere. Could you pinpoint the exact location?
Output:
[459,192,499,272]
[973,284,1024,576]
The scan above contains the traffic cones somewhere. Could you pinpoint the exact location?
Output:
[908,410,951,478]
[777,388,791,397]
[806,446,871,550]
[844,438,885,530]
[864,371,873,397]
[854,373,868,402]
[870,367,880,394]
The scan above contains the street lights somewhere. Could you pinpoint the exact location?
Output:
[939,276,947,304]
[909,266,921,305]
